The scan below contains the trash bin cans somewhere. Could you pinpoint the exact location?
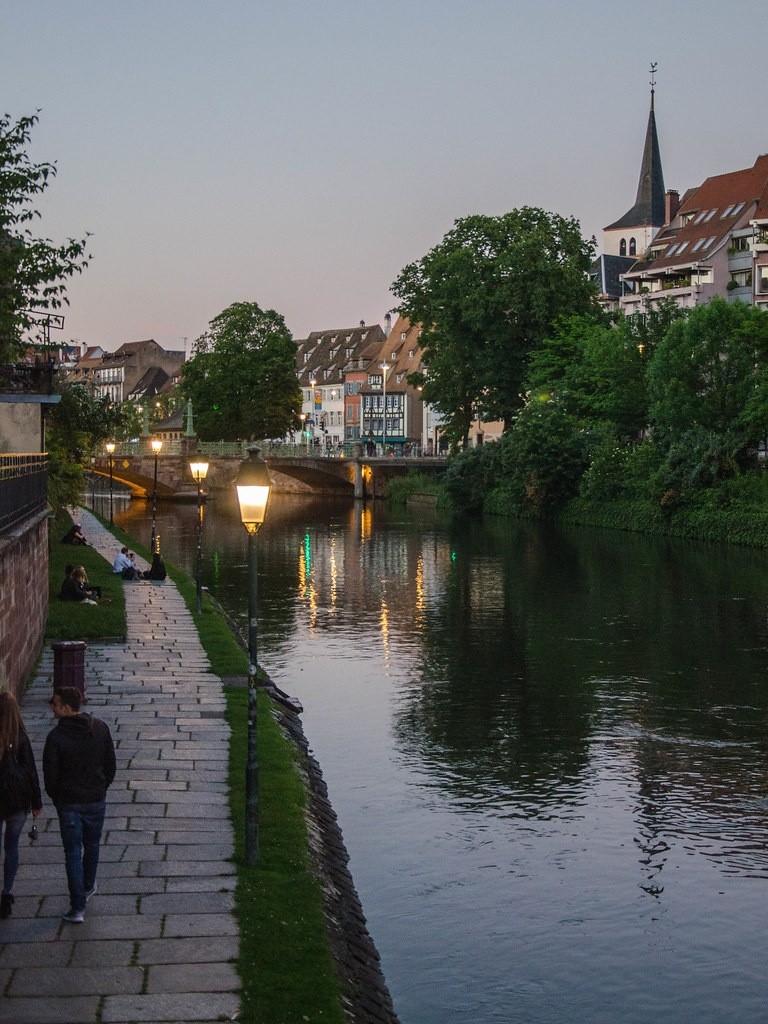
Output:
[49,640,88,705]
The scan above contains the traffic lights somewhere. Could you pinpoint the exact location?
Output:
[319,421,324,431]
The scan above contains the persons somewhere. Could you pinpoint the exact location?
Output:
[310,430,448,458]
[61,525,167,600]
[42,686,117,921]
[0,690,42,919]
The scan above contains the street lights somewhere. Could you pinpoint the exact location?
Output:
[309,378,317,451]
[187,448,210,616]
[105,438,115,527]
[300,414,306,450]
[90,455,96,510]
[150,435,163,554]
[379,358,391,453]
[232,447,275,867]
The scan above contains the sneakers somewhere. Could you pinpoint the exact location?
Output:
[84,880,97,901]
[62,908,85,922]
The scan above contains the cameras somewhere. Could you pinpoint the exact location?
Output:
[29,825,37,839]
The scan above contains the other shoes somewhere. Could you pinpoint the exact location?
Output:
[0,892,15,918]
